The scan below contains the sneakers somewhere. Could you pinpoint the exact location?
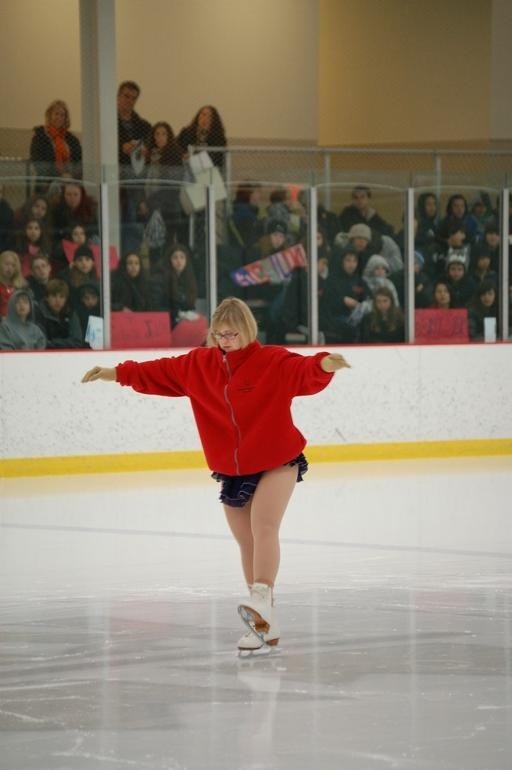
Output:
[238,628,279,650]
[238,598,273,635]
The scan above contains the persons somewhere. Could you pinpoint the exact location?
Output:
[80,294,352,653]
[115,80,155,179]
[29,100,81,193]
[138,120,183,243]
[176,105,227,251]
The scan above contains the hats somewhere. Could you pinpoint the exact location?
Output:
[347,223,371,241]
[268,219,287,233]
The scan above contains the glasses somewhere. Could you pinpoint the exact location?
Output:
[212,332,239,339]
